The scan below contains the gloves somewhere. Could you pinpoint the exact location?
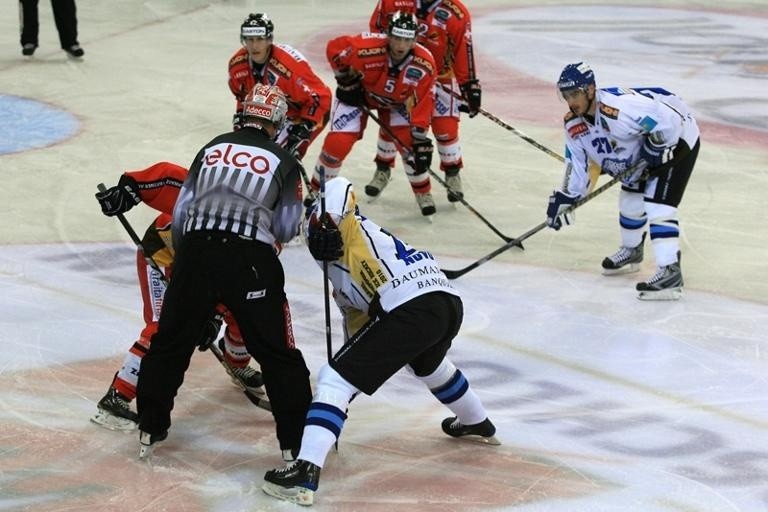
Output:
[96,174,142,218]
[457,79,482,121]
[335,71,368,107]
[196,312,223,353]
[629,160,658,183]
[409,137,434,173]
[546,191,583,232]
[308,226,344,261]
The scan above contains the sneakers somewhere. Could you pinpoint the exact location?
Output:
[263,459,322,492]
[441,415,496,438]
[280,444,299,462]
[636,263,683,292]
[21,42,38,56]
[229,366,264,387]
[138,428,169,445]
[303,192,319,207]
[96,387,138,423]
[65,43,84,58]
[365,168,391,195]
[602,246,644,270]
[415,193,437,217]
[443,168,465,203]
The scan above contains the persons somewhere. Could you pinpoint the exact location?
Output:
[302,18,438,224]
[260,175,502,508]
[136,84,312,468]
[19,1,86,61]
[363,1,481,211]
[228,12,332,246]
[545,62,701,299]
[90,161,273,433]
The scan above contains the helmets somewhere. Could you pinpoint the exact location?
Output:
[386,11,418,50]
[556,61,595,103]
[239,13,273,49]
[243,84,288,130]
